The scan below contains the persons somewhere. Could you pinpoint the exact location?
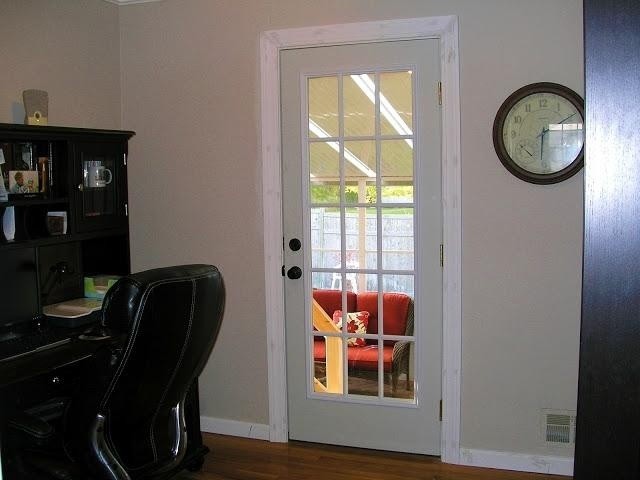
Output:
[26,177,37,193]
[11,171,28,194]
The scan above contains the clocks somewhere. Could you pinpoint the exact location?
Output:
[492,82,586,184]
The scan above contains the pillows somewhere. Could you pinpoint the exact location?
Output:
[331,310,369,348]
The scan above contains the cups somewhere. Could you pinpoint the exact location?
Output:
[87,166,112,188]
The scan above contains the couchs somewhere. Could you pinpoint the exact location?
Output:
[313,288,415,394]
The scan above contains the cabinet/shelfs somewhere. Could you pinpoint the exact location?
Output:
[0,123,136,329]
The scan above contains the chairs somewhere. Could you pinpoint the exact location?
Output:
[3,264,225,480]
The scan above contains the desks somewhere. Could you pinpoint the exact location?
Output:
[1,310,211,480]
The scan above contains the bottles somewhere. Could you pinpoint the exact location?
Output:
[38,158,49,193]
